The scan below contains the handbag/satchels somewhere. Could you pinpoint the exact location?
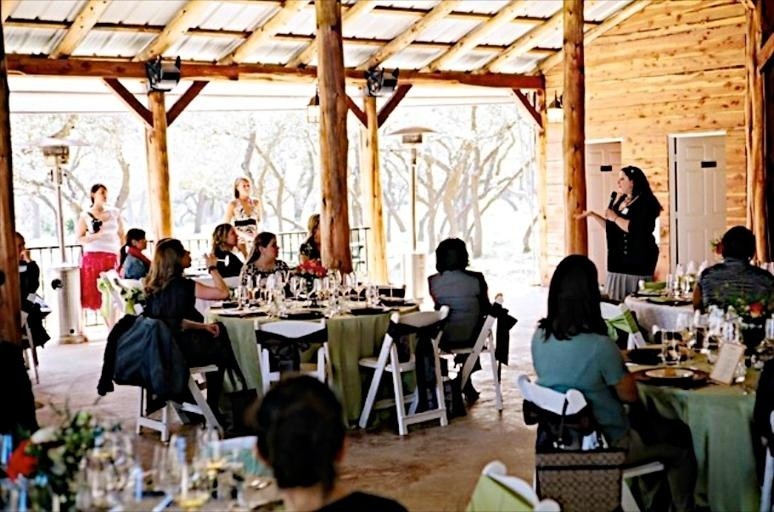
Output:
[429,368,468,424]
[531,444,630,511]
[212,387,259,439]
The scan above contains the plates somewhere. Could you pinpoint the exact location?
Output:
[645,367,695,380]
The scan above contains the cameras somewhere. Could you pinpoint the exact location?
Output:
[92,219,103,233]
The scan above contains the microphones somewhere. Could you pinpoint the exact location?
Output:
[608,191,617,210]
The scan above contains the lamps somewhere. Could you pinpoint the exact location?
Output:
[546,88,565,124]
[142,54,183,93]
[366,67,401,97]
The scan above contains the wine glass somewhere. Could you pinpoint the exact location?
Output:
[659,258,774,383]
[233,268,400,318]
[190,254,230,267]
[0,412,252,512]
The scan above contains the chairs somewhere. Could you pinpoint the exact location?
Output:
[518,374,665,512]
[21,310,39,384]
[357,305,449,436]
[100,271,142,314]
[439,293,505,414]
[464,459,561,512]
[133,365,224,440]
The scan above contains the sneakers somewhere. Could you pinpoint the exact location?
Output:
[464,386,479,408]
